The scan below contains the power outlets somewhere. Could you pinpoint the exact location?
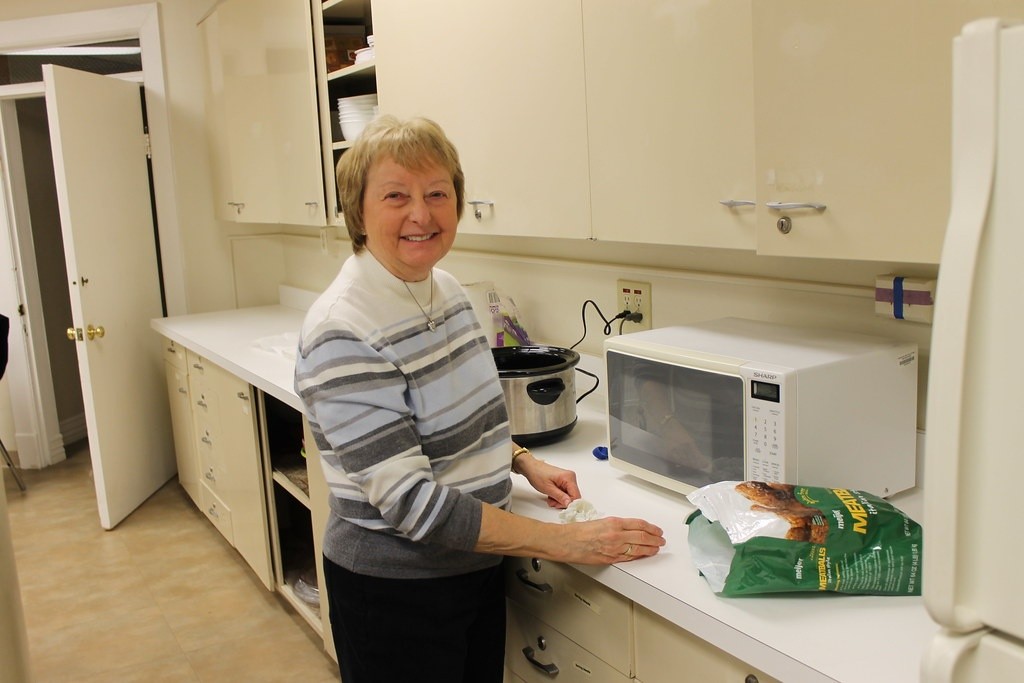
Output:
[616,280,651,334]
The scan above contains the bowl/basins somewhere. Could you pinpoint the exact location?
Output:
[337,93,380,141]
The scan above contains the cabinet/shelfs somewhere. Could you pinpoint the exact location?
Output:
[158,333,777,683]
[195,0,1024,263]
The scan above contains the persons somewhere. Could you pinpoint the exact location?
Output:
[295,112,665,683]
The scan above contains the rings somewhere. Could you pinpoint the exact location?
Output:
[623,544,632,554]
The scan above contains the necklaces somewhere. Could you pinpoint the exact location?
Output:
[403,270,438,332]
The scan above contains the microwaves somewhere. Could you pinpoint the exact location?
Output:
[600,315,918,508]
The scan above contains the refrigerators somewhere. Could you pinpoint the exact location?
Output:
[920,14,1024,683]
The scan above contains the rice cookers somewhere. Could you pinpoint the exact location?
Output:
[489,346,581,447]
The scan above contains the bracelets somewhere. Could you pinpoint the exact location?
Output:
[512,446,529,475]
